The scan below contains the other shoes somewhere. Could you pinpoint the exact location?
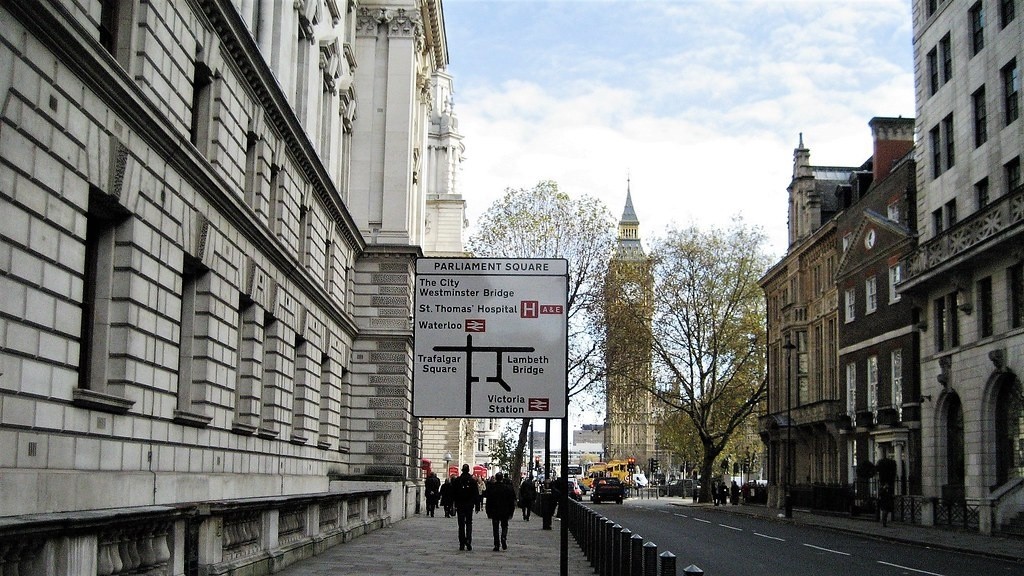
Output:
[459,546,464,551]
[465,541,472,551]
[492,547,499,551]
[501,538,507,550]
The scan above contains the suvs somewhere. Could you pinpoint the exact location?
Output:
[655,479,694,498]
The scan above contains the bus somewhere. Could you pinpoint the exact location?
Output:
[550,459,648,495]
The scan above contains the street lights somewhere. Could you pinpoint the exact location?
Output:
[782,340,797,519]
[442,454,453,482]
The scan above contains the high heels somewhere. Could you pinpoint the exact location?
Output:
[445,514,451,518]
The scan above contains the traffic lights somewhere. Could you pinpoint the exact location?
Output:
[627,458,635,472]
[650,458,659,473]
[536,457,539,467]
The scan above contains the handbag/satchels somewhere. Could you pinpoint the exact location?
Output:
[450,507,457,516]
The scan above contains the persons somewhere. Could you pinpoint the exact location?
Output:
[425,465,561,551]
[624,478,641,497]
[709,480,767,507]
[879,483,894,528]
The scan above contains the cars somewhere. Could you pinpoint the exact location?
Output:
[589,477,624,505]
[568,478,583,501]
